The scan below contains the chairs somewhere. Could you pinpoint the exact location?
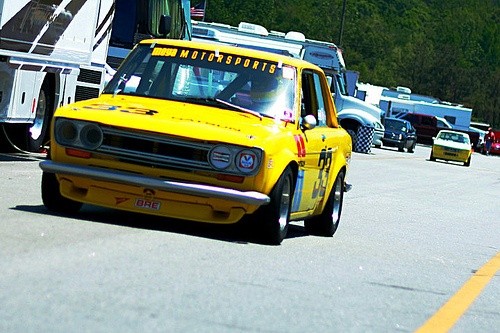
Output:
[458,136,464,142]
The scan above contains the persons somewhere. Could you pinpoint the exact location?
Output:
[242,66,294,117]
[484,128,495,156]
[458,135,464,142]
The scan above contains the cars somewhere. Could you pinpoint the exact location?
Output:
[38,40,353,245]
[394,111,500,156]
[429,130,474,167]
[379,117,417,150]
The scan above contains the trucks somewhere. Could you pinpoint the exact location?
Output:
[154,22,386,149]
[0,0,194,154]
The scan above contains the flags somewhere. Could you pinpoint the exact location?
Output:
[190,1,205,17]
